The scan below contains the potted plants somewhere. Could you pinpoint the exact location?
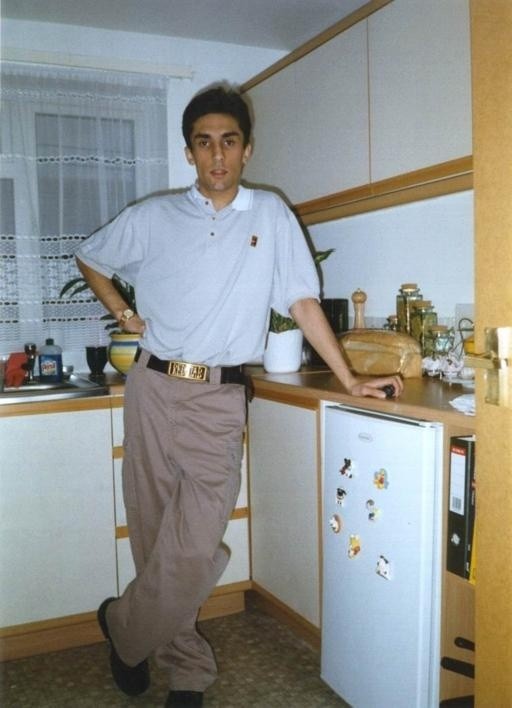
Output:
[57,270,140,376]
[262,248,332,374]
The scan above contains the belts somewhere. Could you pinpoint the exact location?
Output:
[134,348,254,402]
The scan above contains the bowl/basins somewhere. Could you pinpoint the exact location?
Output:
[62,365,73,379]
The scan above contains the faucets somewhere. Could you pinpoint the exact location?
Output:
[21,342,37,381]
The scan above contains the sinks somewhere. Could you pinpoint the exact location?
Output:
[0,379,82,395]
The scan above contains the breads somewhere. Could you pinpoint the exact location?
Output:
[339,329,423,379]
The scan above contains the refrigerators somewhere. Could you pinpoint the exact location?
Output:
[319,406,443,708]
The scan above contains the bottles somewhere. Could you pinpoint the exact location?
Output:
[395,284,452,357]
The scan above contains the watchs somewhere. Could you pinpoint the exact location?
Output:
[119,308,136,326]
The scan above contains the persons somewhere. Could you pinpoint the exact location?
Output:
[74,86,403,708]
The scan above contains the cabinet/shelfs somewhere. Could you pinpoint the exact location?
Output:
[241,3,368,227]
[246,385,323,649]
[368,2,475,214]
[0,394,259,663]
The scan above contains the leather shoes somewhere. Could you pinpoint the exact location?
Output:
[165,691,203,708]
[97,597,151,697]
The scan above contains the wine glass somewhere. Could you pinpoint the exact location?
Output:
[85,347,108,379]
